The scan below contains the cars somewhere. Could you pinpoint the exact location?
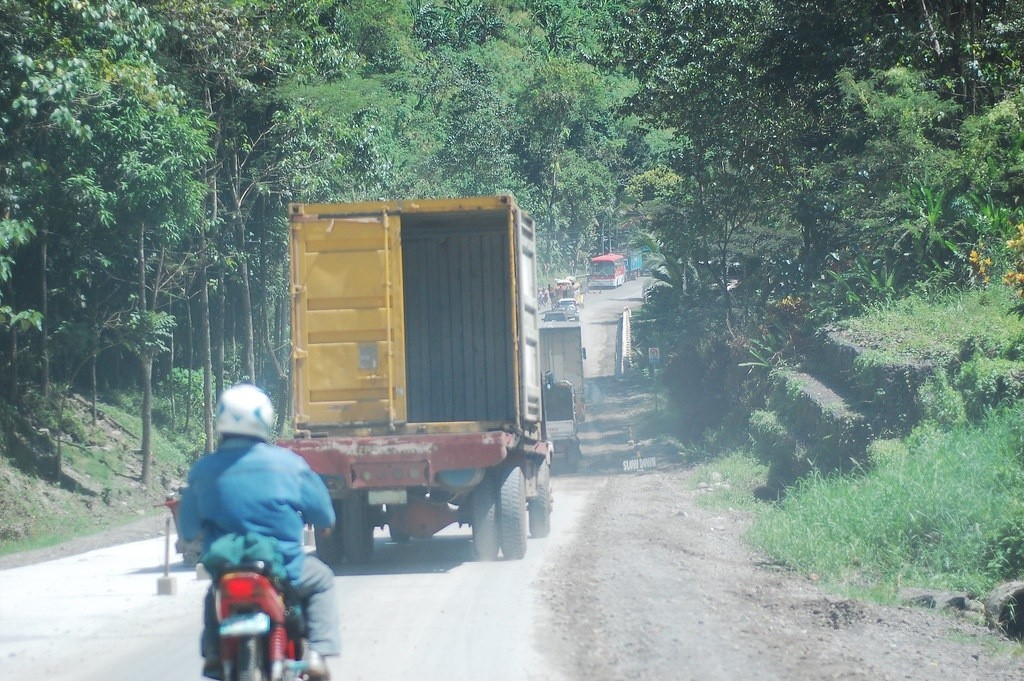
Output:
[541,311,569,323]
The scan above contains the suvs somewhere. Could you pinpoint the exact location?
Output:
[552,297,579,322]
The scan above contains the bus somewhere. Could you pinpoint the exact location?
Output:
[588,252,629,288]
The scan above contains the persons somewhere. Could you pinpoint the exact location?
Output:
[177,384,341,681]
[537,282,576,310]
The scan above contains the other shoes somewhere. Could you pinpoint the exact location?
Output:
[203,651,223,679]
[299,650,329,681]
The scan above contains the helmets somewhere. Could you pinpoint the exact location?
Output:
[216,383,273,443]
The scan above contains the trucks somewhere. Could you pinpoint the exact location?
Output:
[624,247,646,280]
[274,193,575,566]
[540,320,586,397]
[541,371,582,451]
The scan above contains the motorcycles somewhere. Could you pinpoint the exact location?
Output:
[213,511,311,681]
